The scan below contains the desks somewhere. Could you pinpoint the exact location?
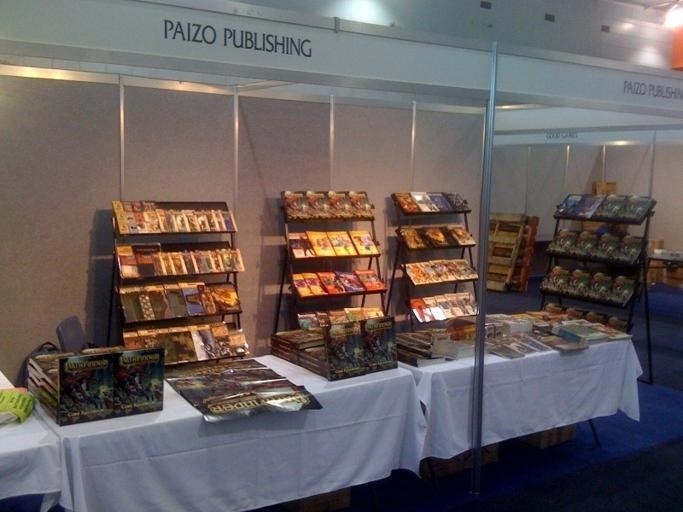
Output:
[398,315,643,477]
[638,255,681,303]
[33,354,428,512]
[1,370,72,512]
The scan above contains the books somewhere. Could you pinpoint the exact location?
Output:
[269,307,399,381]
[280,189,375,223]
[539,265,635,306]
[390,192,472,216]
[286,229,380,258]
[395,226,478,250]
[111,200,237,235]
[114,240,245,278]
[400,258,480,286]
[483,303,632,360]
[485,219,531,283]
[117,281,241,322]
[28,346,164,425]
[290,269,388,297]
[391,325,477,367]
[122,322,251,366]
[547,229,648,264]
[409,292,480,324]
[552,194,652,223]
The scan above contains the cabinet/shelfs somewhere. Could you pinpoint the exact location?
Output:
[106,201,249,372]
[272,190,388,333]
[538,192,657,380]
[386,191,478,313]
[487,211,538,295]
